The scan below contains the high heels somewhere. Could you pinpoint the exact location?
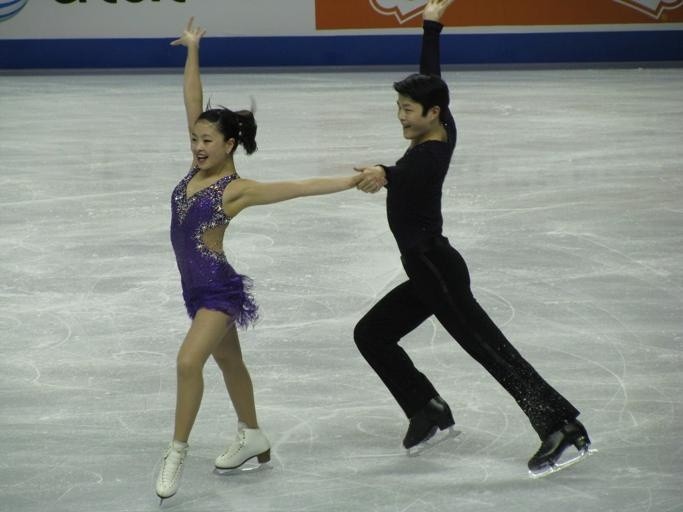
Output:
[528,417,591,471]
[403,394,455,448]
[215,421,271,469]
[155,439,190,499]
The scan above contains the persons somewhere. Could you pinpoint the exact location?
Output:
[153,13,390,501]
[349,0,590,474]
